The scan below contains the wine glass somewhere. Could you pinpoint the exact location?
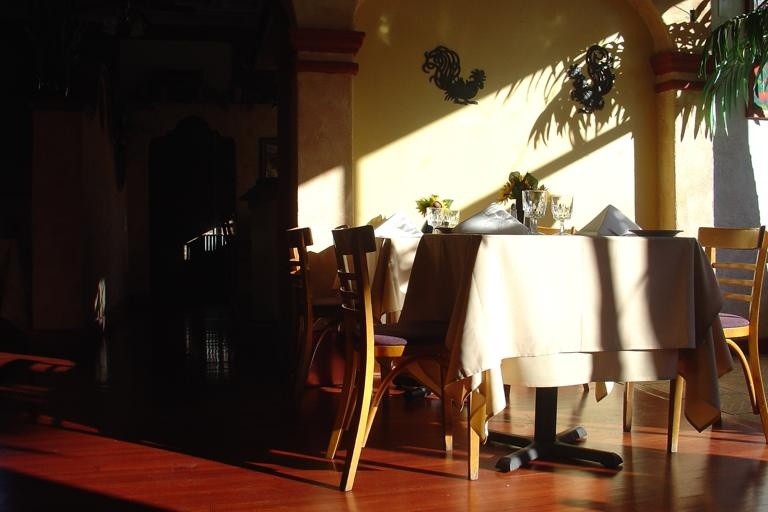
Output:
[426,207,443,235]
[520,189,547,235]
[550,192,574,235]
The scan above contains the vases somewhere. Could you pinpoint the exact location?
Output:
[512,200,524,224]
[419,219,435,235]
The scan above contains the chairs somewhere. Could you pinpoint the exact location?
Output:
[621,222,768,456]
[283,220,412,422]
[325,221,490,492]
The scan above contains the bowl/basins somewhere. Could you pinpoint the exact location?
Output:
[427,209,460,228]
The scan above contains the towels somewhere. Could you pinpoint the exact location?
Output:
[372,208,423,236]
[451,200,547,236]
[573,202,643,236]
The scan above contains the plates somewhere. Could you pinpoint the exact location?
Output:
[627,229,684,238]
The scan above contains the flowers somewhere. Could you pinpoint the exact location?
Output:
[414,191,455,221]
[497,171,553,203]
